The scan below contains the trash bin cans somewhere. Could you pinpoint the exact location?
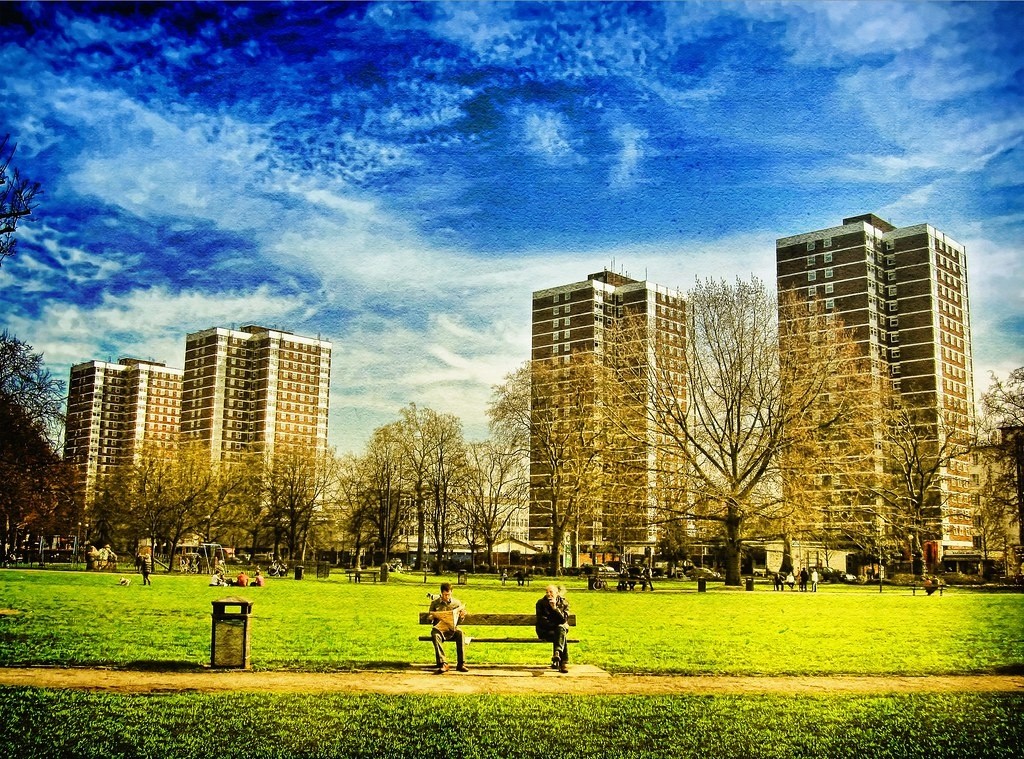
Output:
[207,595,254,669]
[746,577,754,590]
[698,576,706,592]
[295,566,305,580]
[588,574,597,590]
[458,569,467,584]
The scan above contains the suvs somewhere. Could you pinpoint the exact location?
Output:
[753,565,779,577]
[389,558,402,571]
[690,568,720,580]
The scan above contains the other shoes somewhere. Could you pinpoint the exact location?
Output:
[439,664,450,672]
[456,664,468,672]
[560,665,569,673]
[551,653,561,663]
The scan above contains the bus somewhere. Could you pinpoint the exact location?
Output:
[197,548,235,562]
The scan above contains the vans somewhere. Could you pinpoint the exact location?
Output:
[255,554,272,561]
[807,567,834,575]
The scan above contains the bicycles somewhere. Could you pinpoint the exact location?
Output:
[593,577,609,591]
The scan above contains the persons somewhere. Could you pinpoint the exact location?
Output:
[182,554,202,574]
[774,567,818,592]
[210,556,264,587]
[136,547,151,585]
[641,564,655,591]
[925,576,938,595]
[389,563,404,573]
[536,585,570,673]
[513,568,526,586]
[354,563,361,582]
[427,583,469,672]
[500,568,510,584]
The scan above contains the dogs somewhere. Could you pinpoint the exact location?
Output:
[120,577,131,586]
[426,593,441,600]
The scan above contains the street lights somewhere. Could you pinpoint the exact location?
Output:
[908,534,913,582]
[75,522,88,570]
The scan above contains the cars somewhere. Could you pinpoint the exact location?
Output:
[5,550,24,568]
[595,563,614,572]
[228,557,243,565]
[840,570,857,579]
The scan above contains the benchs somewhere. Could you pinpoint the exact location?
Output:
[596,572,648,591]
[501,573,533,586]
[418,613,580,667]
[909,582,947,597]
[774,578,801,591]
[345,569,379,583]
